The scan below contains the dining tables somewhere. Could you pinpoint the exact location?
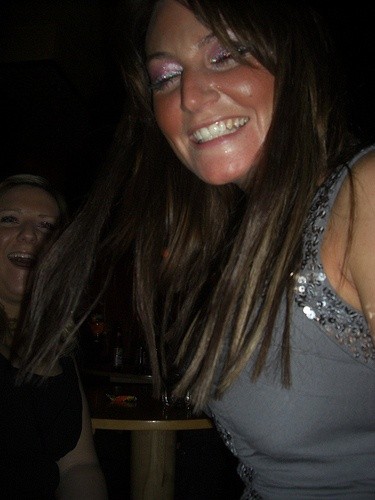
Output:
[81,366,213,500]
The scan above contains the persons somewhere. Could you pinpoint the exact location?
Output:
[0,173,106,500]
[11,1,374,500]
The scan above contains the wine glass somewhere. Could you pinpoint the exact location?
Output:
[90,317,105,347]
